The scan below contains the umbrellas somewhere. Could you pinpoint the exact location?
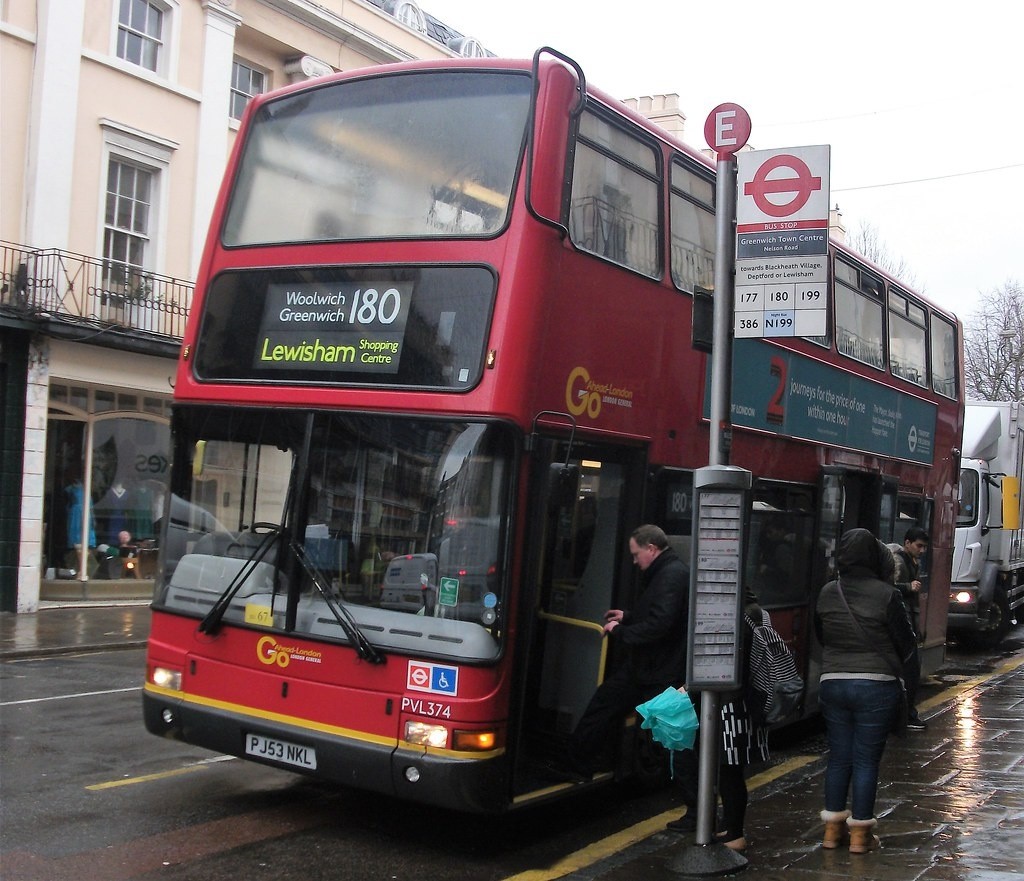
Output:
[635,686,699,778]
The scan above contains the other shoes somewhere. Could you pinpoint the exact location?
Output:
[906,717,928,728]
[666,811,719,830]
[708,820,745,850]
[546,760,593,782]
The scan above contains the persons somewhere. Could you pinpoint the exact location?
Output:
[816,526,928,852]
[66,476,96,581]
[546,517,813,850]
[107,480,155,537]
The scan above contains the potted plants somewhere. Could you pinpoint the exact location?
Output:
[118,268,178,332]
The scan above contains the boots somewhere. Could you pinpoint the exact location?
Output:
[820,809,851,848]
[846,815,881,852]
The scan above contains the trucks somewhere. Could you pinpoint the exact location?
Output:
[949,397,1023,643]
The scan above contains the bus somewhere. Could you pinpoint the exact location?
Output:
[138,46,971,815]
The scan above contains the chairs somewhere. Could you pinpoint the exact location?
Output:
[890,360,900,375]
[900,367,918,383]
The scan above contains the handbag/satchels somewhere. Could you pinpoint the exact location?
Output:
[890,688,907,740]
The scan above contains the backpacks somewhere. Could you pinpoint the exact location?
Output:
[740,606,805,728]
